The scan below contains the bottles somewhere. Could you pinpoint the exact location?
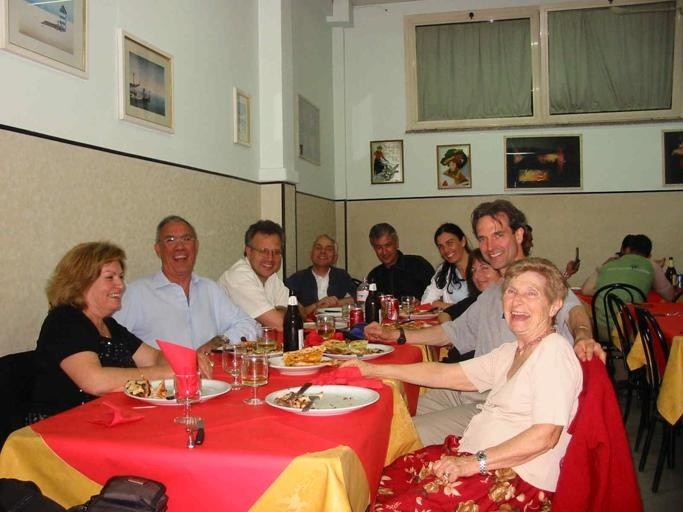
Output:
[281,287,304,352]
[665,255,677,287]
[340,276,382,328]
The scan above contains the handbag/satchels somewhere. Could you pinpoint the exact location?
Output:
[67,475,168,512]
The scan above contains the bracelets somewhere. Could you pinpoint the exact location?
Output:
[574,323,593,339]
[136,366,147,380]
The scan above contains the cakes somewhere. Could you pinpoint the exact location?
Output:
[284,345,325,367]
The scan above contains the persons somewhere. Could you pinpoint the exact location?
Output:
[111,216,266,366]
[361,198,608,364]
[218,219,308,338]
[436,247,501,324]
[440,147,469,188]
[284,233,358,312]
[595,234,682,347]
[581,234,636,297]
[367,222,436,302]
[372,145,389,177]
[419,223,470,307]
[26,241,212,425]
[338,256,585,512]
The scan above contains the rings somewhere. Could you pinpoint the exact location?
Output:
[444,472,451,477]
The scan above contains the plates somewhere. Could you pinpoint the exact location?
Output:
[398,308,440,321]
[315,305,346,316]
[263,383,379,418]
[265,340,394,377]
[124,377,232,407]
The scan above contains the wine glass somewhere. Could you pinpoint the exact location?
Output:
[170,371,202,425]
[400,295,414,329]
[221,343,268,407]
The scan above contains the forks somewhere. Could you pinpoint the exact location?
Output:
[184,416,196,448]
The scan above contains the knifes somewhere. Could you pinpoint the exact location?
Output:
[195,416,205,444]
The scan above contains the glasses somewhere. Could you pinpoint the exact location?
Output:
[250,246,281,256]
[162,237,194,243]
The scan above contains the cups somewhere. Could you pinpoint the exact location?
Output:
[316,314,336,343]
[255,325,277,353]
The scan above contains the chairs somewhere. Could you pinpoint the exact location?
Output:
[0,352,41,453]
[555,281,674,511]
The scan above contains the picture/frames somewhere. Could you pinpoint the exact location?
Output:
[119,31,176,135]
[437,144,472,189]
[660,130,683,188]
[503,133,584,190]
[1,0,88,82]
[369,139,405,184]
[231,88,252,147]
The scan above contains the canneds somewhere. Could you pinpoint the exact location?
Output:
[379,294,399,321]
[350,308,364,330]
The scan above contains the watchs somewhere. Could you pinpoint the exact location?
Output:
[474,450,489,473]
[397,327,406,345]
[218,335,230,344]
